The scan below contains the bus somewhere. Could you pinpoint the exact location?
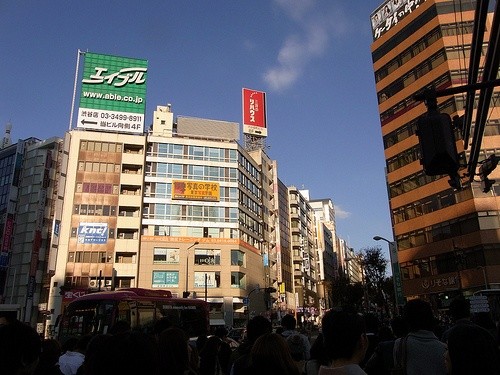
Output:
[473,289,500,330]
[53,288,211,375]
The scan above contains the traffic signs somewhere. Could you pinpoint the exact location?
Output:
[469,296,488,300]
[76,107,144,135]
[470,300,489,304]
[471,304,490,308]
[470,308,491,312]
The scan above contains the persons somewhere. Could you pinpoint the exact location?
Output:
[0,299,500,375]
